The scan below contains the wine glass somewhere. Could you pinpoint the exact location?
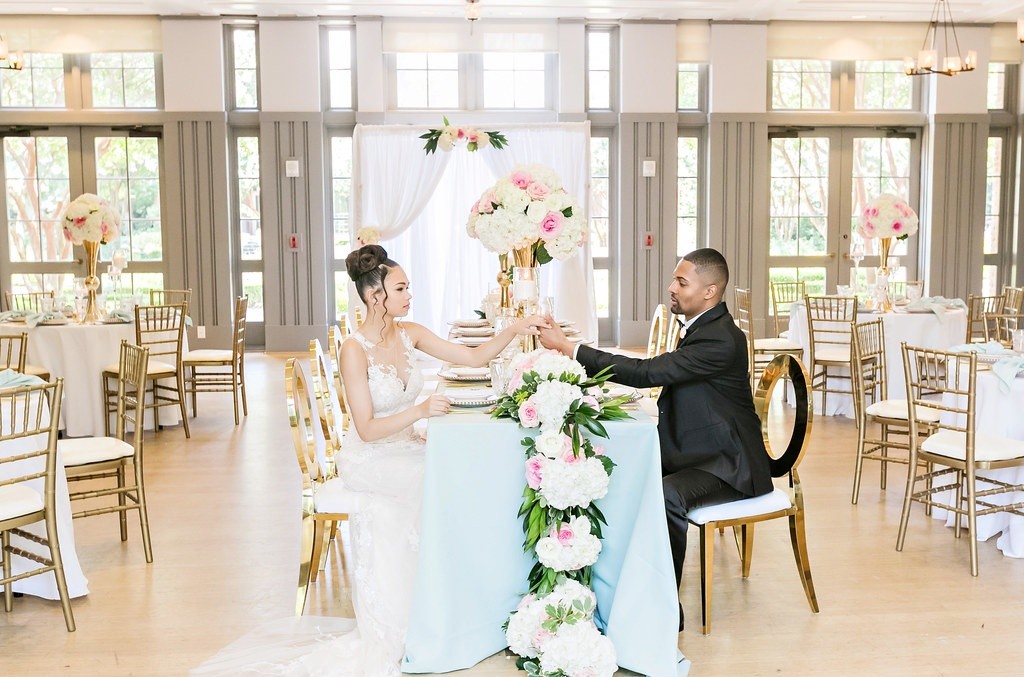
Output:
[488,360,514,412]
[849,243,864,272]
[74,299,88,326]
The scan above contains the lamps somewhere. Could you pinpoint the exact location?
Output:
[1016,16,1024,43]
[906,0,977,76]
[0,37,24,70]
[465,0,481,22]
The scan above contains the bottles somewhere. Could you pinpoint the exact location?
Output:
[494,307,521,359]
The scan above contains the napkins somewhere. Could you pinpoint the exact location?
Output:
[0,369,65,398]
[444,313,638,402]
[0,303,193,327]
[811,295,1024,394]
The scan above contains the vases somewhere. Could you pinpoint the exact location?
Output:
[79,238,104,324]
[877,238,893,313]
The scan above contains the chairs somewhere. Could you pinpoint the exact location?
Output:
[981,313,1024,348]
[895,342,1024,577]
[993,283,1024,342]
[4,290,56,312]
[150,288,192,333]
[965,293,1008,343]
[892,280,927,301]
[284,308,352,618]
[849,317,941,516]
[58,340,152,563]
[647,281,878,635]
[102,302,191,440]
[182,295,249,425]
[0,331,28,374]
[0,377,76,632]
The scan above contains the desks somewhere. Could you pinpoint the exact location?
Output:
[0,318,194,437]
[402,326,692,677]
[931,356,1024,559]
[1,388,90,600]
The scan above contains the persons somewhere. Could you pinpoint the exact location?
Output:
[335,244,552,661]
[537,248,773,633]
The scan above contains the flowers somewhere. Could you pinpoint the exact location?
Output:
[855,193,918,240]
[358,227,381,246]
[679,326,686,339]
[60,193,122,247]
[419,116,510,154]
[492,348,640,677]
[464,165,590,265]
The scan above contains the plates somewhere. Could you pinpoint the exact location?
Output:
[906,310,933,313]
[447,319,490,327]
[145,317,172,320]
[437,368,493,380]
[977,355,1012,364]
[554,318,575,327]
[450,337,492,346]
[8,317,27,321]
[103,319,127,324]
[566,337,593,344]
[38,320,68,324]
[441,389,498,407]
[559,327,580,335]
[450,329,493,337]
[856,308,878,313]
[605,388,643,403]
[894,299,910,306]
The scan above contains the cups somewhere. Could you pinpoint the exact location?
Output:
[53,296,66,312]
[1011,329,1024,353]
[837,285,855,306]
[120,295,142,312]
[40,298,53,315]
[74,278,88,298]
[909,286,922,308]
